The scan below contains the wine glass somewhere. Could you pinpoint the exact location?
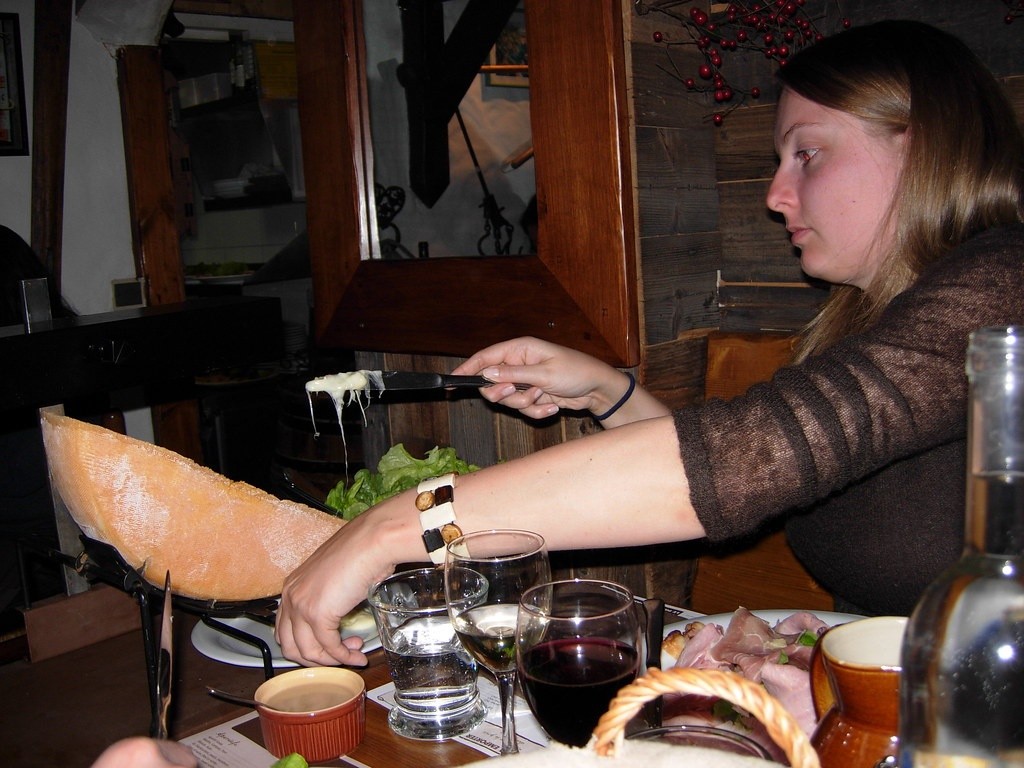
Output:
[445,529,552,757]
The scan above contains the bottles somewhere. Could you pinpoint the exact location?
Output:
[898,322,1024,768]
[810,616,910,768]
[226,40,258,99]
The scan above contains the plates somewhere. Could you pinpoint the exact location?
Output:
[187,271,255,285]
[662,610,871,672]
[211,178,250,198]
[190,600,381,668]
[194,321,307,385]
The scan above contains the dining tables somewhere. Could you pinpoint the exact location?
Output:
[0,599,685,768]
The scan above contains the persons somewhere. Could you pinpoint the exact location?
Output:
[272,17,1024,665]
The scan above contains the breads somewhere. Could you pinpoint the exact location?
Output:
[39,410,350,603]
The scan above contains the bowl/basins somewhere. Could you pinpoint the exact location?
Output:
[253,667,366,764]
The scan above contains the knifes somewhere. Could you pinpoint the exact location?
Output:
[154,569,172,741]
[306,371,530,391]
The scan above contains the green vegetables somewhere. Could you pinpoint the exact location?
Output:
[713,630,819,732]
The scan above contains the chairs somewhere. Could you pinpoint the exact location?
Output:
[678,328,836,616]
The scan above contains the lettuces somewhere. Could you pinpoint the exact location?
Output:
[269,751,309,768]
[325,444,508,519]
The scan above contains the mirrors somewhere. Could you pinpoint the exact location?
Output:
[292,0,639,372]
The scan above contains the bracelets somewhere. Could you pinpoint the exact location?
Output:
[415,469,471,565]
[594,372,635,421]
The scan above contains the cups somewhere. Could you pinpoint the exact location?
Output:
[370,570,490,742]
[203,73,232,103]
[516,581,642,755]
[178,76,204,110]
